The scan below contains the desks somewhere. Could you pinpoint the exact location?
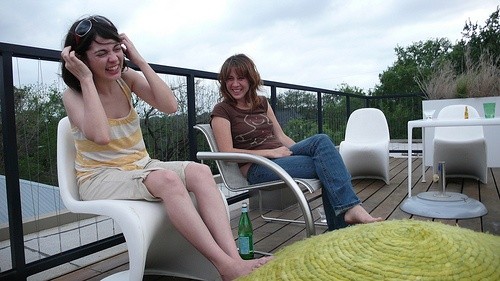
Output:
[408,118,500,198]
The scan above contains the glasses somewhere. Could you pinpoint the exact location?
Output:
[74,15,114,36]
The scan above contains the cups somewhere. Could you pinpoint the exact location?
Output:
[483,102,496,118]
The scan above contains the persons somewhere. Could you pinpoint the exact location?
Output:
[60,16,274,281]
[209,54,382,231]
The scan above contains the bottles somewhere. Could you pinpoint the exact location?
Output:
[238,202,254,259]
[464,106,468,119]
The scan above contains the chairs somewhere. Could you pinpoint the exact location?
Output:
[57,116,231,281]
[340,108,390,185]
[193,124,329,256]
[433,105,487,184]
